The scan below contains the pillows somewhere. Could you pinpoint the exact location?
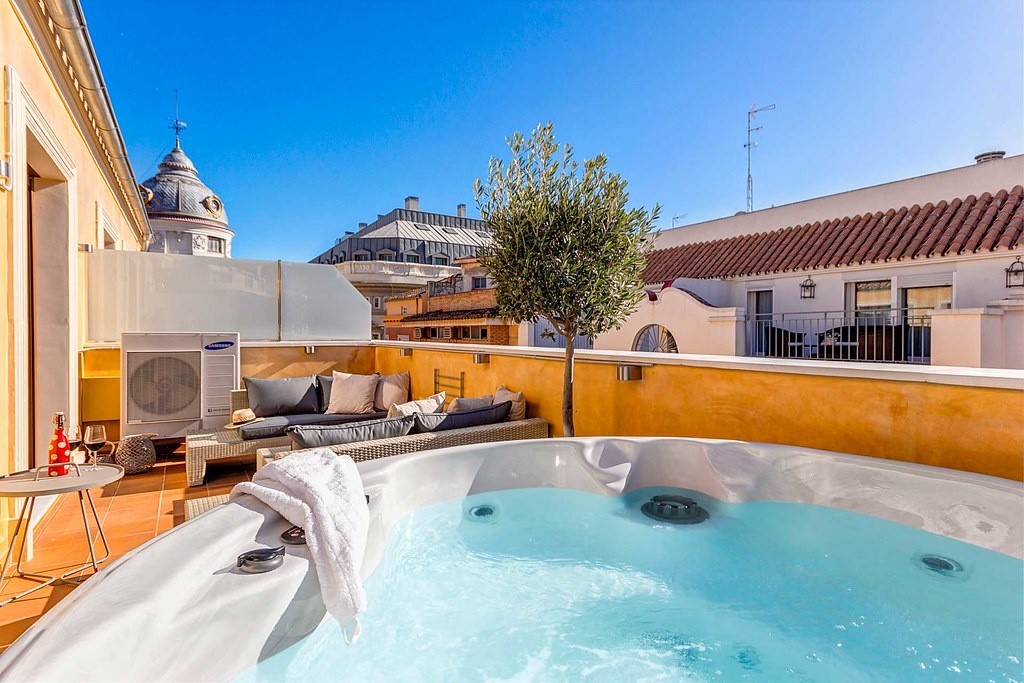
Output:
[241,371,526,451]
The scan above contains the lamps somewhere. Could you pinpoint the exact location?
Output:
[0,159,8,180]
[400,347,412,356]
[799,275,816,297]
[305,346,315,354]
[1004,256,1024,287]
[616,365,642,381]
[80,244,93,253]
[473,354,489,363]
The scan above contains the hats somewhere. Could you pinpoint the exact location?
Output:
[223,407,265,429]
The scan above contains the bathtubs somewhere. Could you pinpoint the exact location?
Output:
[0,436,1024,682]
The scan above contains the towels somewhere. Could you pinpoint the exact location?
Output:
[228,446,372,648]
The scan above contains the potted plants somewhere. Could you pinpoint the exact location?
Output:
[824,332,841,344]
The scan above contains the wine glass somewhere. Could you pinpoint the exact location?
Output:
[83,425,107,472]
[61,425,82,471]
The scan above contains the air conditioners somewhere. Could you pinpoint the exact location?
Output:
[119,331,240,437]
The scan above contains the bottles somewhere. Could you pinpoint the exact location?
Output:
[48,412,71,477]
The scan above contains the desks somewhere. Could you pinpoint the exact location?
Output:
[0,462,124,606]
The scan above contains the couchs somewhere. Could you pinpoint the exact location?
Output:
[184,390,290,487]
[765,326,807,357]
[185,417,548,521]
[814,325,908,361]
